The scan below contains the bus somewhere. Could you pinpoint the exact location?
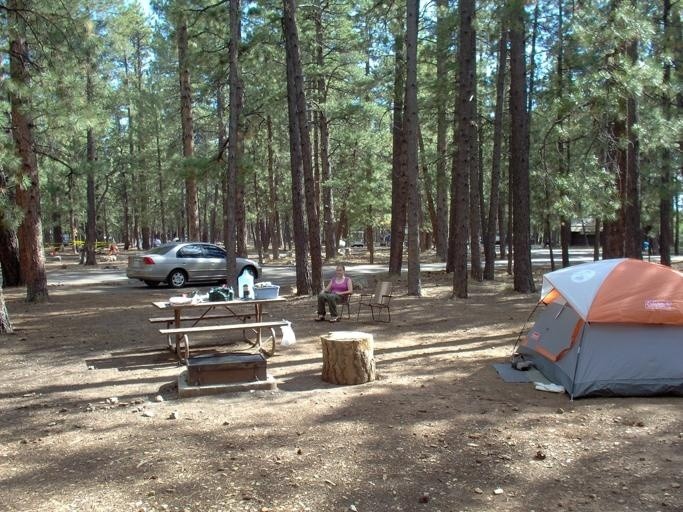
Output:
[350,230,364,247]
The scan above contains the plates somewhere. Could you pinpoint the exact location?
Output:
[169,296,191,304]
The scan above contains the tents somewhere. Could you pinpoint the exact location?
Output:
[511,257,683,401]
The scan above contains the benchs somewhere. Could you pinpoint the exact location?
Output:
[159,320,289,365]
[149,310,268,352]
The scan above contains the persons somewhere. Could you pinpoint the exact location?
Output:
[152,235,160,248]
[314,265,353,322]
[109,243,119,256]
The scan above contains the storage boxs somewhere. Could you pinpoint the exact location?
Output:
[253,285,280,300]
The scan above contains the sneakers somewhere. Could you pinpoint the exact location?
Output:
[329,317,339,322]
[315,316,326,321]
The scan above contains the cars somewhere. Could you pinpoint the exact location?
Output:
[127,242,262,285]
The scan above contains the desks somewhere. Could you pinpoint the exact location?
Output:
[152,294,288,356]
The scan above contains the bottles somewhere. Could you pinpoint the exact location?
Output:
[243,284,249,300]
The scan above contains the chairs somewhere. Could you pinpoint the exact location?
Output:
[357,278,394,323]
[317,281,353,322]
